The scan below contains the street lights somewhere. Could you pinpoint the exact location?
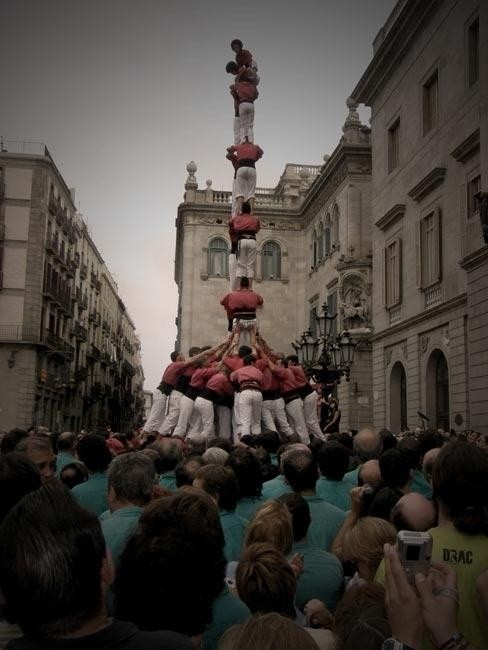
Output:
[290,298,361,435]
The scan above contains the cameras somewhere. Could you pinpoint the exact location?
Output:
[396,531,433,598]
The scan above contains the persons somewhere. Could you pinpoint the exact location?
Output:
[143,323,341,445]
[1,426,487,648]
[221,37,264,322]
